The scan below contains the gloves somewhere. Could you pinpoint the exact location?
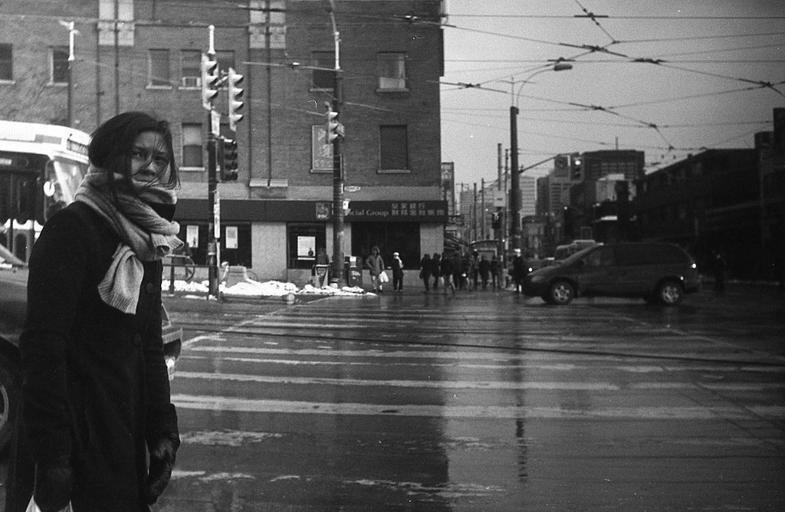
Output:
[145,432,181,505]
[34,466,72,512]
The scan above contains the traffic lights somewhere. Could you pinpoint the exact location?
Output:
[228,66,245,133]
[200,52,221,113]
[570,157,584,183]
[219,135,239,183]
[326,109,340,145]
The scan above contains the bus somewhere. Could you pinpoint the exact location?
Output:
[0,119,94,264]
[554,239,605,261]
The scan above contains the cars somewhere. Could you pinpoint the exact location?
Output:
[0,238,188,442]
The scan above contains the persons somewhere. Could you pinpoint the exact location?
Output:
[20,112,185,510]
[307,241,524,294]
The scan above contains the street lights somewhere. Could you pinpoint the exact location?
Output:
[509,64,575,259]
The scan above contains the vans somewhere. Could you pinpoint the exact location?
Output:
[517,238,703,306]
